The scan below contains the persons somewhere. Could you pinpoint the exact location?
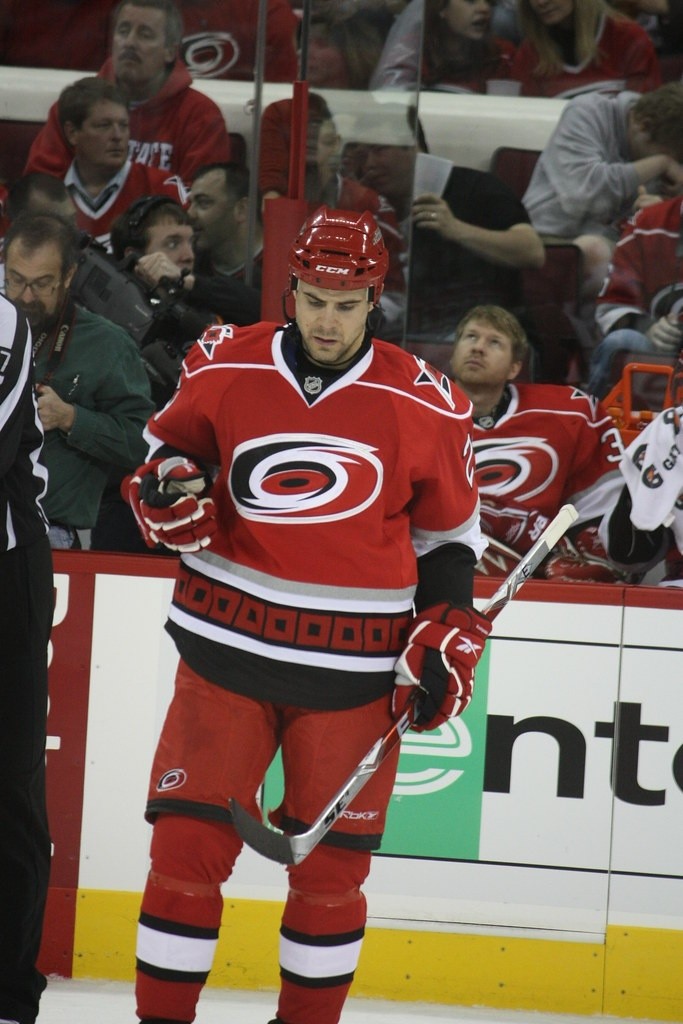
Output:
[1,290,53,1023]
[0,1,683,587]
[117,206,493,1023]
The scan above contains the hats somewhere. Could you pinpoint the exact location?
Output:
[286,205,388,308]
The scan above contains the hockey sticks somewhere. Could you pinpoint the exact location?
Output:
[232,503,584,866]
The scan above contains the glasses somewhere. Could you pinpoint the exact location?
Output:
[4,276,61,298]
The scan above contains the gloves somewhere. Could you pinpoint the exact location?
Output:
[391,599,494,730]
[115,453,218,553]
